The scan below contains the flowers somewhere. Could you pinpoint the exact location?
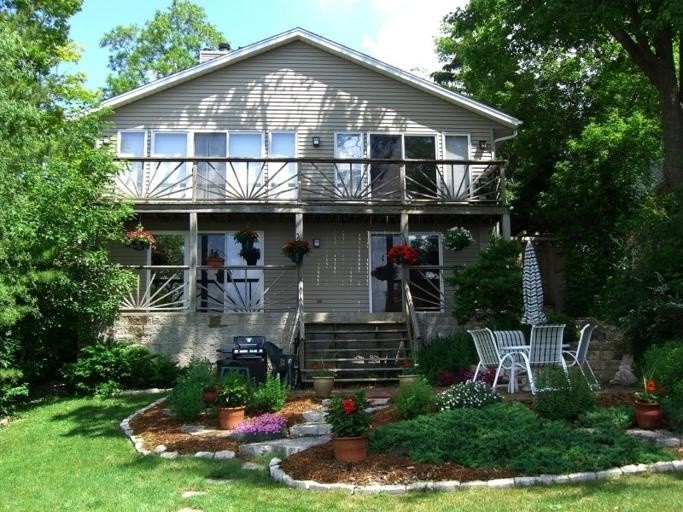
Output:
[324,386,377,437]
[124,230,158,250]
[444,226,475,251]
[396,356,423,375]
[234,222,259,241]
[388,244,416,263]
[634,365,668,403]
[280,240,310,257]
[241,248,261,258]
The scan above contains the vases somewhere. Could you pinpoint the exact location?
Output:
[454,242,464,251]
[635,400,663,430]
[398,375,419,386]
[246,258,257,265]
[392,260,403,280]
[134,241,146,251]
[240,238,254,250]
[330,435,369,463]
[292,249,303,263]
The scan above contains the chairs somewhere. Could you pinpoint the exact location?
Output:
[563,323,602,391]
[510,322,572,396]
[492,329,527,371]
[466,327,519,393]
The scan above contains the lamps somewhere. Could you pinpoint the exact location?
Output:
[313,137,320,145]
[313,238,321,247]
[480,139,487,150]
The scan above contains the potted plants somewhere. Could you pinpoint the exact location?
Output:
[213,366,250,429]
[311,349,338,397]
[205,248,224,265]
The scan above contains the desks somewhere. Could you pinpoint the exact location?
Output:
[499,344,570,394]
